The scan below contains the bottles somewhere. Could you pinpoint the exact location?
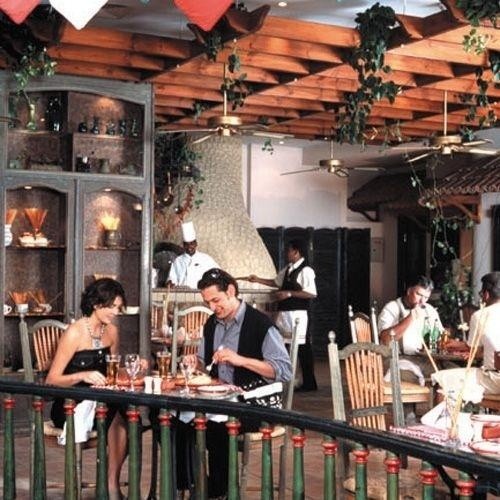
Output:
[143,376,162,395]
[423,317,441,355]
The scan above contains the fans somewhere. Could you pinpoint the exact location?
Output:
[391,91,500,164]
[279,127,387,179]
[158,65,294,143]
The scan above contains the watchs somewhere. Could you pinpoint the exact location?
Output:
[249,239,318,392]
[286,290,292,298]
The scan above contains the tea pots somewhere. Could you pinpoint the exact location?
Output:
[18,232,52,248]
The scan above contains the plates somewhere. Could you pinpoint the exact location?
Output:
[470,439,500,461]
[199,386,230,396]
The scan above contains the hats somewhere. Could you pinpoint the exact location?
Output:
[181,221,196,242]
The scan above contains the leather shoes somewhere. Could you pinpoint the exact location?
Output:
[293,383,318,392]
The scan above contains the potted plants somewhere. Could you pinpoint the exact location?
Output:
[156,132,206,209]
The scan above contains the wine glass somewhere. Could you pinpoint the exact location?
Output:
[124,354,141,392]
[179,354,197,399]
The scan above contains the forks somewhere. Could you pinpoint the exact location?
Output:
[203,344,225,376]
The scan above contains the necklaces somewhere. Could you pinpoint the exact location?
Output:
[84,317,105,348]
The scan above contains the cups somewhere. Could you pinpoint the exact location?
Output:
[156,351,171,382]
[105,355,121,387]
[3,304,52,315]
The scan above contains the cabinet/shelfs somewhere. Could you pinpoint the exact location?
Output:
[0,68,154,438]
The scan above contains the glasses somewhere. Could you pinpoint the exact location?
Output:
[207,272,221,280]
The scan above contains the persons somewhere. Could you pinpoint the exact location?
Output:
[166,238,221,290]
[378,273,460,421]
[467,272,500,401]
[176,269,294,500]
[45,276,148,500]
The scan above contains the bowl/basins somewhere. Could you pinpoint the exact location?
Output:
[121,306,140,315]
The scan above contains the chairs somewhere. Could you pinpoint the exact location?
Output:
[17,300,499,498]
